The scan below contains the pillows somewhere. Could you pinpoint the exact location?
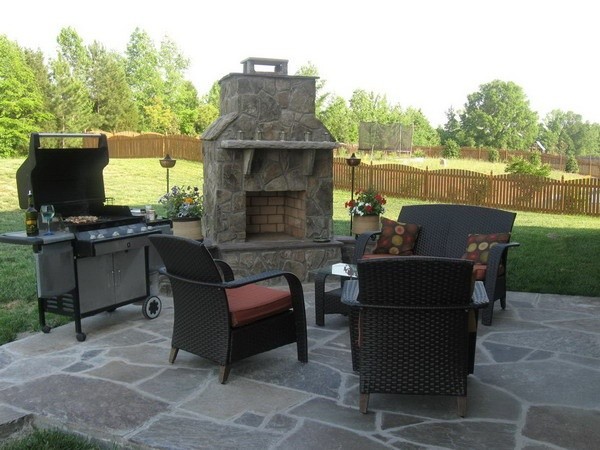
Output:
[459,232,511,279]
[372,216,422,255]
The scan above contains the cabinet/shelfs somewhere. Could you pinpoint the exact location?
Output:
[76,248,149,316]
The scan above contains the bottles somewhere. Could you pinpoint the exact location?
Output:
[25,190,39,236]
[147,210,155,221]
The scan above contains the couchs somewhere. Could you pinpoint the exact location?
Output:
[316,204,518,326]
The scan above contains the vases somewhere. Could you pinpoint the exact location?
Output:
[171,219,203,242]
[351,215,380,234]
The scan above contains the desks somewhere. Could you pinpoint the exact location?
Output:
[340,280,490,367]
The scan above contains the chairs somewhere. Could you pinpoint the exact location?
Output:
[347,255,490,417]
[153,235,307,385]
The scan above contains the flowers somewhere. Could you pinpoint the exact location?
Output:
[158,185,205,219]
[345,188,386,217]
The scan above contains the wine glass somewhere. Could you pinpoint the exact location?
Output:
[40,204,55,236]
